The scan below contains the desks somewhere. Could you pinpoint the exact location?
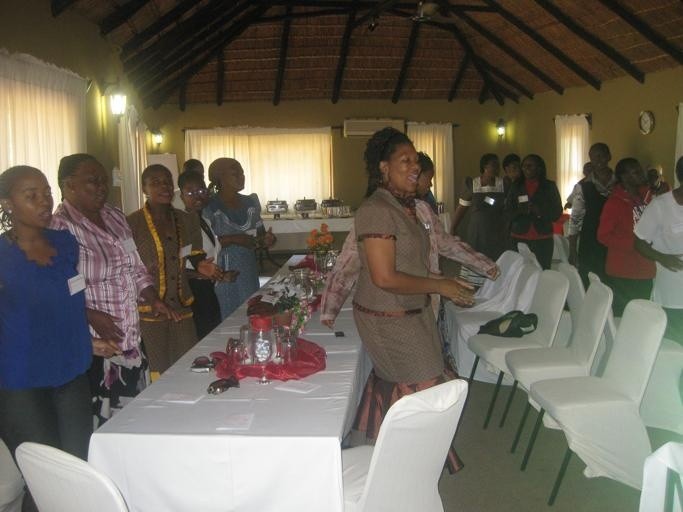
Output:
[260,212,355,267]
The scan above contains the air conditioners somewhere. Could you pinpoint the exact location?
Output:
[344,117,405,139]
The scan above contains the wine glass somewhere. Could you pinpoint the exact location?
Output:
[247,327,277,384]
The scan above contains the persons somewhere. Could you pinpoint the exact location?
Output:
[0,166,123,512]
[320,151,502,329]
[352,128,477,474]
[125,164,191,386]
[451,153,562,270]
[48,153,182,434]
[169,157,276,338]
[563,144,682,343]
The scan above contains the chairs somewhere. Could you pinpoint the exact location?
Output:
[341,377,469,512]
[15,440,128,512]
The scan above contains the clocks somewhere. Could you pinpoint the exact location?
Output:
[638,110,656,135]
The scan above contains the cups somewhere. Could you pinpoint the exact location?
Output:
[217,248,232,271]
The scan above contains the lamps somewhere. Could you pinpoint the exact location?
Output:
[496,118,506,145]
[151,128,163,153]
[102,77,126,115]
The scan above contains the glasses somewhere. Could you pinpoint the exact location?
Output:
[183,189,208,198]
